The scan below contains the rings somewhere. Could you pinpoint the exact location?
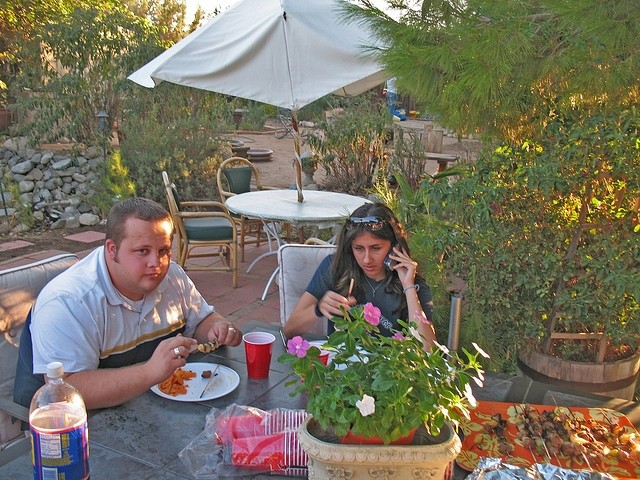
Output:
[174,347,180,357]
[229,329,237,334]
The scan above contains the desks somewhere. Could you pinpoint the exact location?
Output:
[223,188,375,300]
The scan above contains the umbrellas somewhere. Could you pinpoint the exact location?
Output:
[125,0,396,204]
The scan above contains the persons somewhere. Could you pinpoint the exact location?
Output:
[282,203,438,357]
[11,196,243,413]
[381,76,407,122]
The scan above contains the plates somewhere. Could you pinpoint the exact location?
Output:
[307,340,370,370]
[150,362,240,403]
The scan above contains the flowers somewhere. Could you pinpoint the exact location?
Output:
[277,301,490,446]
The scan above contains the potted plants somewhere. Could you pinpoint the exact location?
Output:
[456,104,639,401]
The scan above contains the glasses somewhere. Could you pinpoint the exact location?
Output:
[345,215,389,232]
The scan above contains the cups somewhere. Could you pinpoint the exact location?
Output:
[242,332,276,380]
[231,431,309,477]
[215,408,309,445]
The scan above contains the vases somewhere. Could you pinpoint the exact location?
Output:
[294,414,463,479]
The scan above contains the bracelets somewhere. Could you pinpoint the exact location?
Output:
[402,283,420,296]
[314,299,323,319]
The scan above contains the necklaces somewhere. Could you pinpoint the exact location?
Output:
[362,270,390,298]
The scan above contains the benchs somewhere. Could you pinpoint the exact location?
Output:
[381,146,461,174]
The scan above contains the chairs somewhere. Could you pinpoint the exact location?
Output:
[0,254,81,447]
[160,170,238,290]
[217,155,290,262]
[276,242,340,334]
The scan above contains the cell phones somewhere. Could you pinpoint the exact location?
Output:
[383,243,401,272]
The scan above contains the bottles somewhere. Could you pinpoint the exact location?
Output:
[28,362,90,480]
